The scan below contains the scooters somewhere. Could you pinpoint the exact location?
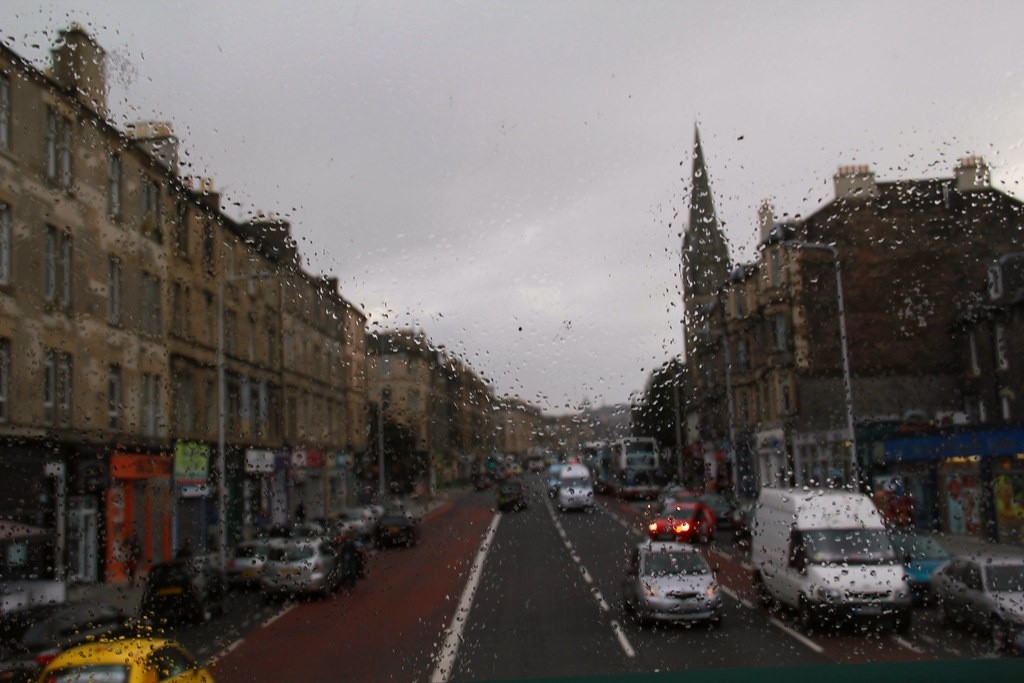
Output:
[490,471,525,513]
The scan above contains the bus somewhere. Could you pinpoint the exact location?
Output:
[578,437,660,500]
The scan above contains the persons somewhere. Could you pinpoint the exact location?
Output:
[122,530,141,587]
[177,537,193,558]
[295,503,307,522]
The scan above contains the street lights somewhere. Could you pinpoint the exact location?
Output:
[776,238,858,497]
[695,329,740,504]
[216,271,271,576]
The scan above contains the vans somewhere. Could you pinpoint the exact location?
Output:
[547,464,594,514]
[752,485,913,633]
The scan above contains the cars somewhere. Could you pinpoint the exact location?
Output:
[230,501,420,595]
[649,484,754,550]
[622,541,721,631]
[1,601,212,683]
[888,531,1024,652]
